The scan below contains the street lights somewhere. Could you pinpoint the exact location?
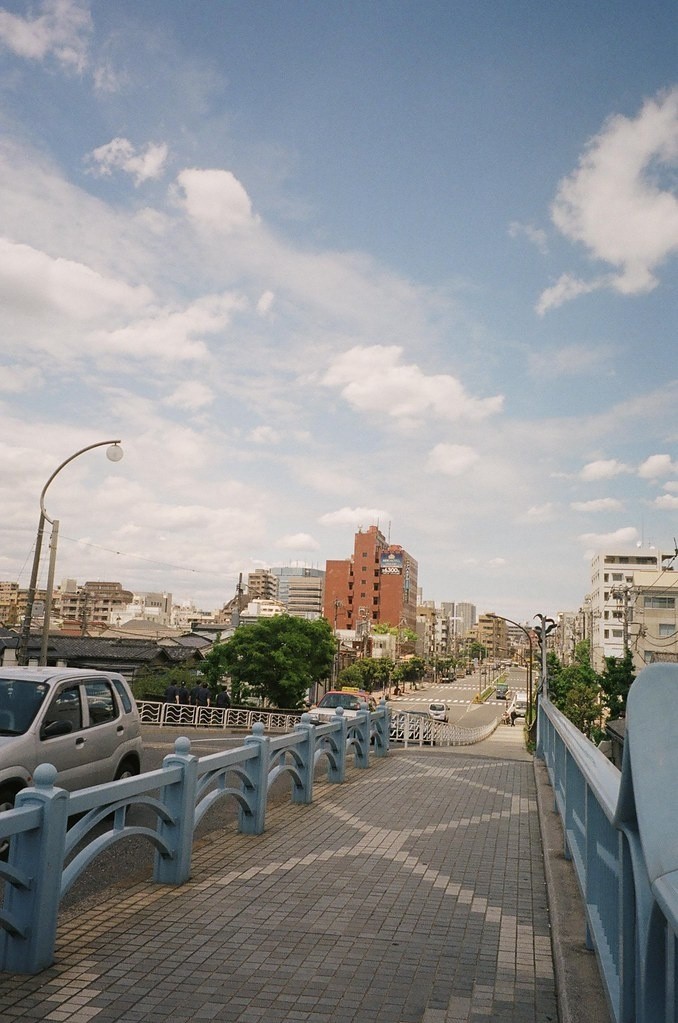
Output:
[38,438,124,668]
[486,614,533,728]
[500,647,529,716]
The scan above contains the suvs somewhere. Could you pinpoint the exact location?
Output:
[0,665,142,861]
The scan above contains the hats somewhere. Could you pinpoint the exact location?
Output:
[181,680,186,686]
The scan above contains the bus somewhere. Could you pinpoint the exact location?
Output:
[512,691,529,717]
[496,683,508,700]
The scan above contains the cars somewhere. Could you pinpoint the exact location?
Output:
[440,656,518,684]
[389,711,436,745]
[304,687,381,745]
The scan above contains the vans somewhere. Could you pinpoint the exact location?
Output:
[429,703,450,724]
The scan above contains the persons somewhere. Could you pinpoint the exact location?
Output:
[164,680,179,704]
[216,686,230,728]
[188,679,202,726]
[510,710,516,726]
[194,682,212,727]
[171,679,191,726]
